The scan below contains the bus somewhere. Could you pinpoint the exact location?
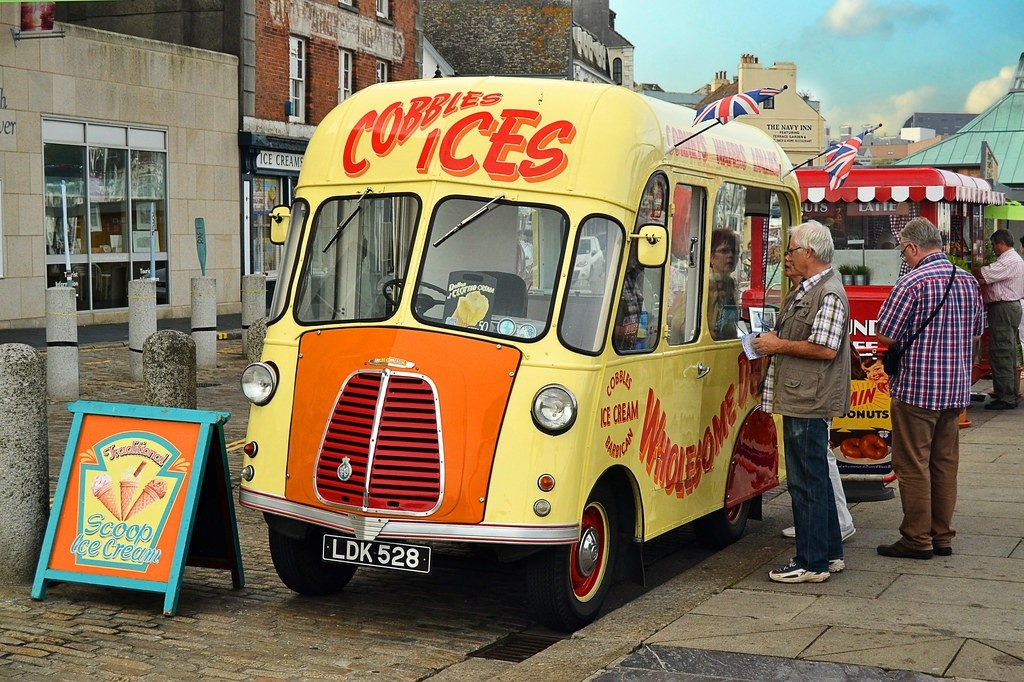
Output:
[736,166,1006,388]
[239,75,805,633]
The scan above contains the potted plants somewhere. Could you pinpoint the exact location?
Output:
[838,264,870,286]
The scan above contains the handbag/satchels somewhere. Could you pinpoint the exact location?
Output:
[881,349,901,376]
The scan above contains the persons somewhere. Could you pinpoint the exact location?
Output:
[873,218,988,560]
[710,228,740,341]
[615,216,655,350]
[751,218,857,581]
[667,255,687,344]
[971,228,1023,409]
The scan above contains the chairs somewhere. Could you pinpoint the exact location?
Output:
[462,271,529,319]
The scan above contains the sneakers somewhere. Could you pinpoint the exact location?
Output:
[877,541,953,560]
[782,526,795,537]
[985,401,1017,410]
[841,524,857,542]
[768,557,846,583]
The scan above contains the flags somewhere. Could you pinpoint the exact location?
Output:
[823,129,868,189]
[692,87,781,129]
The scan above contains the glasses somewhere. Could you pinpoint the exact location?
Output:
[715,246,740,255]
[900,244,918,262]
[784,246,802,257]
